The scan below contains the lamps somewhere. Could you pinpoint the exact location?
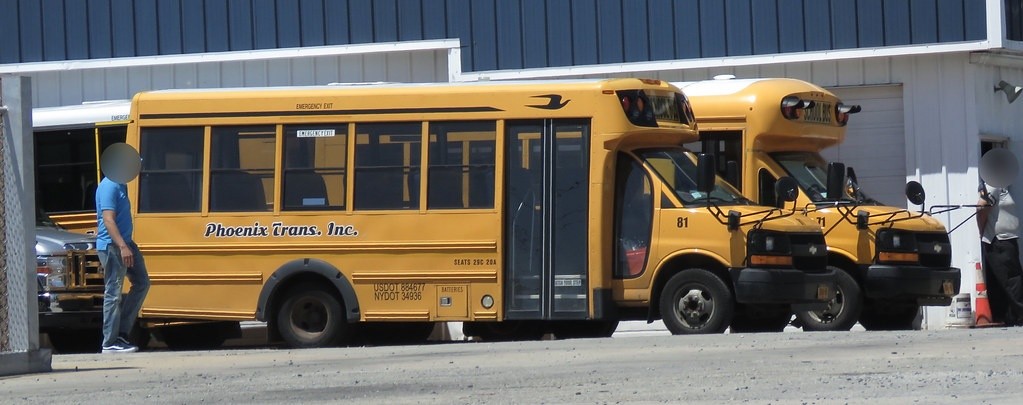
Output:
[994,80,1023,104]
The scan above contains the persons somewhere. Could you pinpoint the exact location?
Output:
[96,176,149,353]
[977,182,1023,326]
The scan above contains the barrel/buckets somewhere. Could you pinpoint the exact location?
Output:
[944,291,975,329]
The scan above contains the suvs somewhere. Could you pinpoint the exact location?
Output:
[32,206,104,342]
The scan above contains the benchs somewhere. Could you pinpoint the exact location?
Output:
[283,171,328,210]
[138,174,195,213]
[210,173,266,212]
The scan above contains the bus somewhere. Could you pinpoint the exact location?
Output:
[33,98,241,348]
[667,78,962,331]
[121,79,831,348]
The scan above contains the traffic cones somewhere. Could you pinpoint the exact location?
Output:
[972,263,992,328]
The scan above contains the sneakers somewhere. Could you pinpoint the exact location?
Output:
[101,336,141,354]
